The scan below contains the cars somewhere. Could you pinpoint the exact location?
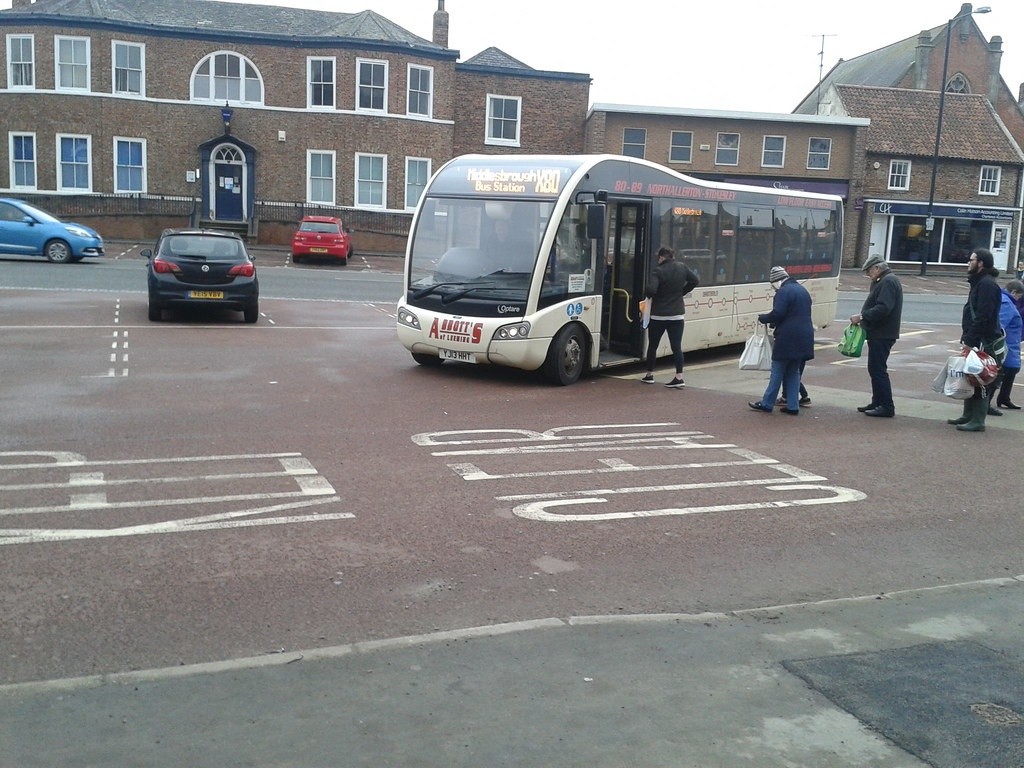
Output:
[0,197,106,264]
[290,215,353,266]
[139,225,260,323]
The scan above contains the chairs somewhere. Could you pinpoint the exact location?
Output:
[677,224,733,250]
[213,241,231,255]
[173,239,188,250]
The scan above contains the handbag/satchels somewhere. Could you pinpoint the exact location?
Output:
[928,356,948,395]
[984,327,1009,368]
[837,322,867,358]
[962,344,998,386]
[946,356,975,400]
[739,318,773,372]
[638,297,652,329]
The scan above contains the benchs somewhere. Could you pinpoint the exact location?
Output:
[675,249,729,287]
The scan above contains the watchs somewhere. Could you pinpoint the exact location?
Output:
[860,315,863,320]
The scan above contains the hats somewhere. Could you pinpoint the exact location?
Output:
[861,253,885,271]
[770,266,789,283]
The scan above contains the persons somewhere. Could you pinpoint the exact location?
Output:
[483,219,529,272]
[1016,259,1024,283]
[850,254,903,418]
[948,249,1002,432]
[987,280,1024,416]
[641,246,699,387]
[748,266,815,415]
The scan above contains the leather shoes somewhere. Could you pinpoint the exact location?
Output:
[748,401,772,413]
[779,407,799,415]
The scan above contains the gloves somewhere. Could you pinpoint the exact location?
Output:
[758,314,766,324]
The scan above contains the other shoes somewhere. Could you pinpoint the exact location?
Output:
[864,406,894,417]
[948,416,970,425]
[857,404,875,412]
[957,419,985,431]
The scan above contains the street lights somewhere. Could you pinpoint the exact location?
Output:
[920,6,992,277]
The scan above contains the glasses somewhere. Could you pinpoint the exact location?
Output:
[969,258,974,262]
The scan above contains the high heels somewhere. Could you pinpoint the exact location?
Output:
[996,395,1021,409]
[987,405,1004,416]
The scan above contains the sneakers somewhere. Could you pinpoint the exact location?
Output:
[774,396,787,406]
[663,377,686,388]
[799,397,812,406]
[641,374,655,383]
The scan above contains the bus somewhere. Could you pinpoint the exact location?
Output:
[395,153,844,388]
